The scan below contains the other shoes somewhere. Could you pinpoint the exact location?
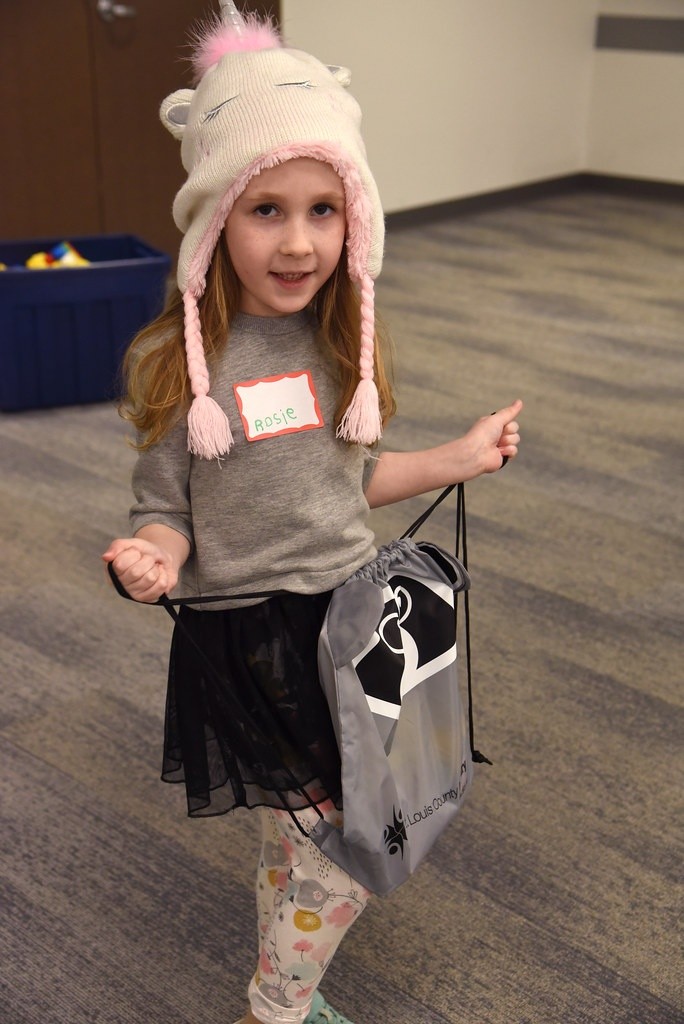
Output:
[305,989,354,1024]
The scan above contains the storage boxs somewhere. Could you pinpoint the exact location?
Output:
[0,233,173,414]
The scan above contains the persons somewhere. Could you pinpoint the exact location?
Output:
[101,0,525,1024]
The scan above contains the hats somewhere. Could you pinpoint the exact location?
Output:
[161,9,384,458]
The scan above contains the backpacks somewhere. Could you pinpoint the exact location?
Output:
[108,412,493,898]
